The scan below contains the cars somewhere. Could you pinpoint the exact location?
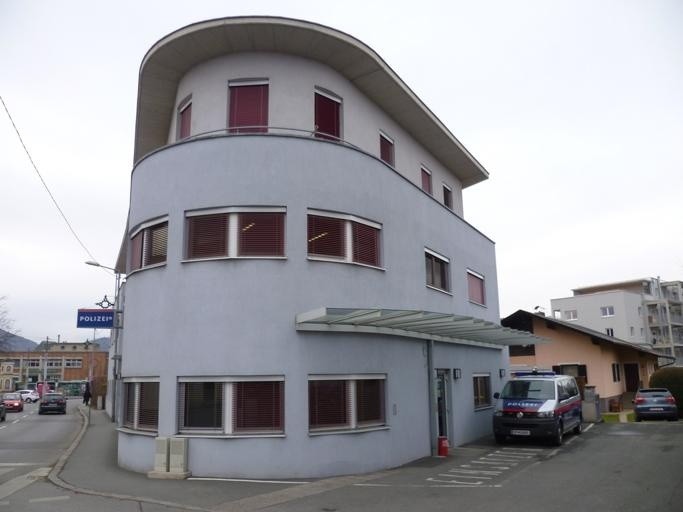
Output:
[15,389,39,403]
[630,386,678,422]
[0,399,6,422]
[0,392,23,412]
[37,391,66,415]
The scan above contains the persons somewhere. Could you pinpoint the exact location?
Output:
[83,388,92,406]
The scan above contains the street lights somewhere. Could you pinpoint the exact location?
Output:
[83,255,122,423]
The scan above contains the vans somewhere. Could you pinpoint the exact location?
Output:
[489,368,584,448]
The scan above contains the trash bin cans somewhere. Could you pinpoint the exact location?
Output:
[609,399,623,412]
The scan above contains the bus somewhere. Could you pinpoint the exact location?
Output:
[56,380,88,397]
